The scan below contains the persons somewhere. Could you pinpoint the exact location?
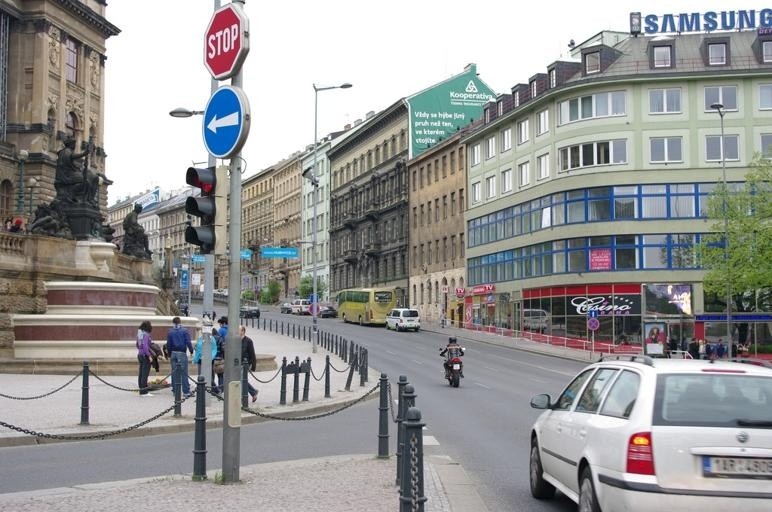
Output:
[54,138,88,204]
[191,334,220,392]
[217,316,229,340]
[123,203,153,255]
[166,317,194,397]
[440,309,447,328]
[440,336,465,379]
[240,326,258,409]
[664,334,739,361]
[211,328,226,387]
[136,321,154,395]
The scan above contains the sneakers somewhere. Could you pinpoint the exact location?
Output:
[140,392,258,401]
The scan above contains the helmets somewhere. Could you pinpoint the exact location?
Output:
[448,336,457,344]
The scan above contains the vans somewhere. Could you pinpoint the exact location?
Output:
[291,299,311,315]
[517,309,548,333]
[384,308,421,332]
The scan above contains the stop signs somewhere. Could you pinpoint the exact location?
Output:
[203,2,250,81]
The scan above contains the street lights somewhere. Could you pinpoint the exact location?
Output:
[710,103,733,359]
[168,108,205,118]
[312,82,353,354]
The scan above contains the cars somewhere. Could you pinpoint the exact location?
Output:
[280,302,290,314]
[529,354,772,512]
[240,305,260,318]
[320,306,338,318]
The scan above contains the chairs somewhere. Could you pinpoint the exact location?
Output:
[591,376,771,421]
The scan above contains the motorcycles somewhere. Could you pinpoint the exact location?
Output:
[437,346,467,387]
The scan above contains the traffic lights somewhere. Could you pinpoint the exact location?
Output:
[185,166,227,253]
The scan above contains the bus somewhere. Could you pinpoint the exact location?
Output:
[336,288,397,326]
